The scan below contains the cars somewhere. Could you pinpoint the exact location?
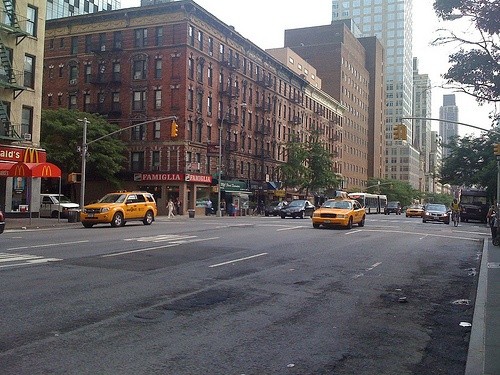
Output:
[312,191,366,230]
[196,196,291,217]
[406,204,425,218]
[280,199,315,219]
[421,203,451,224]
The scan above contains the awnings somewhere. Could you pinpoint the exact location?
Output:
[267,182,278,190]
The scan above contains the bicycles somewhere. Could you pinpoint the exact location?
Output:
[453,211,459,228]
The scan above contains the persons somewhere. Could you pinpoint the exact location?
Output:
[227,201,234,217]
[166,195,181,218]
[205,199,212,216]
[16,177,23,188]
[486,205,500,242]
[257,200,265,216]
[450,198,462,227]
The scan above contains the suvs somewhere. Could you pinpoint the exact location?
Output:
[40,193,80,219]
[384,200,402,215]
[79,189,159,226]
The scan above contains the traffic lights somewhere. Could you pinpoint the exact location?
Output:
[494,143,500,156]
[393,124,400,140]
[171,120,178,138]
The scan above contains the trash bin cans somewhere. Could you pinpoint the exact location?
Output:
[188,211,195,218]
[67,211,78,223]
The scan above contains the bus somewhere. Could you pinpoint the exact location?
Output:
[348,192,387,214]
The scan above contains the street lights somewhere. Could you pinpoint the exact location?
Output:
[217,102,248,218]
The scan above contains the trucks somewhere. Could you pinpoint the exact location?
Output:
[458,188,493,223]
